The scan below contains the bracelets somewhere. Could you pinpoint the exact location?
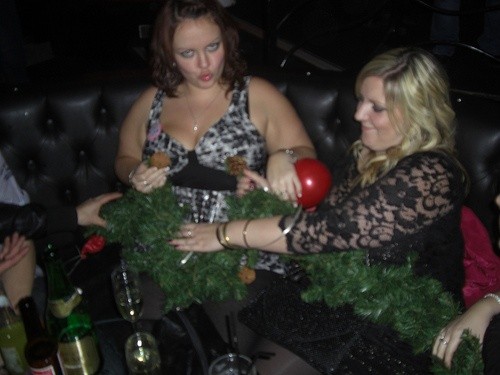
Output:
[243,217,253,250]
[216,220,236,251]
[277,149,298,165]
[483,292,500,304]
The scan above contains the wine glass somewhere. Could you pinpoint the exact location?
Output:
[111,265,145,332]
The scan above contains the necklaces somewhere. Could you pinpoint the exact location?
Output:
[182,84,222,131]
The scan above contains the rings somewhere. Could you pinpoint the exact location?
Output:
[437,334,444,339]
[186,230,191,237]
[439,339,448,345]
[280,191,288,195]
[148,183,153,189]
[143,180,149,186]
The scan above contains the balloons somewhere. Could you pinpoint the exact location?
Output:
[293,158,330,208]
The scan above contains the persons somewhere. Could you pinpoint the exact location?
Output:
[0,192,122,313]
[113,1,318,225]
[431,192,500,368]
[167,46,468,374]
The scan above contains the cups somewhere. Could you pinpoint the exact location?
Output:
[208,353,257,375]
[124,333,163,375]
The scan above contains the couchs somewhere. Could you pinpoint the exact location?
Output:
[0,71,500,256]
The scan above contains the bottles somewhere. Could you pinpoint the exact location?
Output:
[0,295,29,375]
[17,296,62,375]
[40,242,104,375]
